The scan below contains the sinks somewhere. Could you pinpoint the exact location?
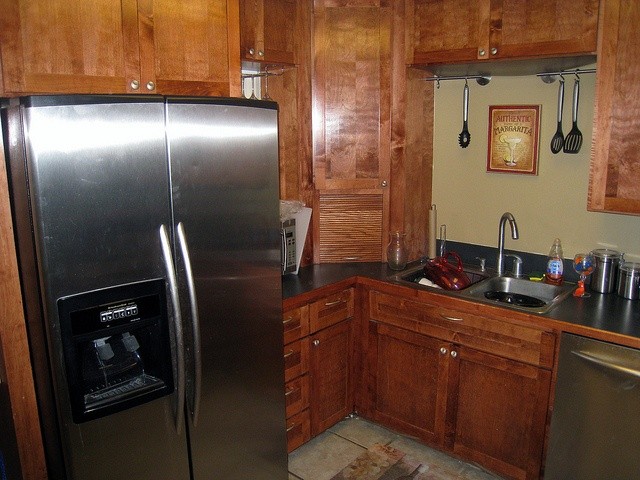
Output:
[449,275,578,314]
[384,258,496,293]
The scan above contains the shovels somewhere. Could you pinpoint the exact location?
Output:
[563,81,583,154]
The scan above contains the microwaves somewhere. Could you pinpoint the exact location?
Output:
[279,199,312,277]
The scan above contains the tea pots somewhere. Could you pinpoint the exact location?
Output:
[423,252,471,291]
[387,231,414,270]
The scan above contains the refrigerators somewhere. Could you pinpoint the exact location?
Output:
[0,92,289,480]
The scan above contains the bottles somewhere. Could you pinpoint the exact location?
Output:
[546,238,565,286]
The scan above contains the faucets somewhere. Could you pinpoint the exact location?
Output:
[494,211,518,274]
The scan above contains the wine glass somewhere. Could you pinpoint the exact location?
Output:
[573,253,596,298]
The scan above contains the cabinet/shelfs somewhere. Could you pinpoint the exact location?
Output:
[240,1,295,72]
[1,1,241,99]
[406,0,599,76]
[262,0,434,265]
[354,277,562,480]
[587,1,640,216]
[283,278,356,454]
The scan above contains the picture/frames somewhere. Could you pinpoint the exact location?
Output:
[486,104,541,177]
